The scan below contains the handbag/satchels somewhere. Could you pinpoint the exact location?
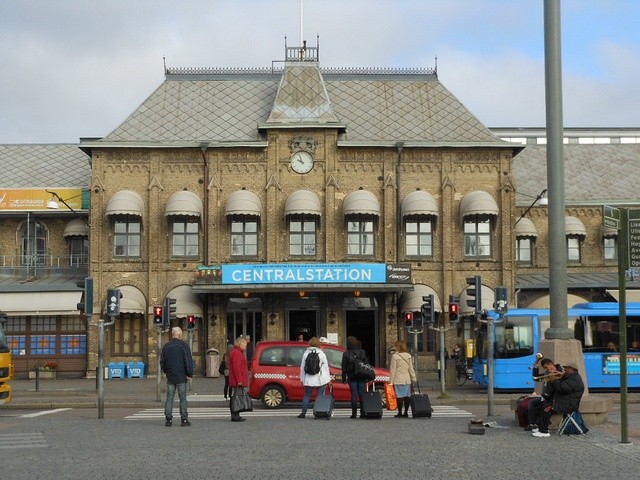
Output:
[384,383,398,411]
[346,358,375,380]
[219,354,227,375]
[230,385,251,412]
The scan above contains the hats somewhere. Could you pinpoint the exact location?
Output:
[319,337,328,343]
[562,362,578,370]
[346,336,358,346]
[227,344,234,351]
[535,352,544,364]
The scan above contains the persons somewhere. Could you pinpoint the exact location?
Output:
[532,361,585,438]
[297,337,331,419]
[524,358,564,431]
[341,337,372,418]
[389,340,417,418]
[452,344,465,383]
[436,347,449,382]
[223,344,234,399]
[228,337,250,422]
[297,335,304,342]
[160,327,194,427]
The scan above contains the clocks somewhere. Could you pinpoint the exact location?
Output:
[290,151,314,174]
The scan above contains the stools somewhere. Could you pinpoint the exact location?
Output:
[557,410,584,436]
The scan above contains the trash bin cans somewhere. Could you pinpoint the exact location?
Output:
[206,348,219,377]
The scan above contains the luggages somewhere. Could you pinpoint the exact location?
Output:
[313,384,335,420]
[360,379,382,419]
[411,380,432,419]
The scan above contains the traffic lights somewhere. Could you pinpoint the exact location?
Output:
[449,304,459,322]
[466,274,481,313]
[165,297,176,325]
[187,315,195,329]
[153,306,163,326]
[449,295,460,303]
[76,278,93,316]
[405,312,413,328]
[422,293,434,322]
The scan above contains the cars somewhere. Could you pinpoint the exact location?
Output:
[249,342,392,409]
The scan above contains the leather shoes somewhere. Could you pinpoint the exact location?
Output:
[402,412,408,417]
[395,412,402,417]
[231,416,246,421]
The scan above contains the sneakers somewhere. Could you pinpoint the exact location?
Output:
[166,418,172,426]
[182,419,191,426]
[531,427,539,432]
[524,424,536,431]
[532,431,550,438]
[298,413,305,418]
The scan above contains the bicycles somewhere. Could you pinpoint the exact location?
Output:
[456,359,474,386]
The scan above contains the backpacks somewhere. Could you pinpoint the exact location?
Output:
[304,349,324,374]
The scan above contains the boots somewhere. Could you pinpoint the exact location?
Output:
[350,400,357,419]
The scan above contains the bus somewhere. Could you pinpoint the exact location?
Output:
[471,302,640,393]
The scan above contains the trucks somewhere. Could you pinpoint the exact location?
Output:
[0,311,14,405]
